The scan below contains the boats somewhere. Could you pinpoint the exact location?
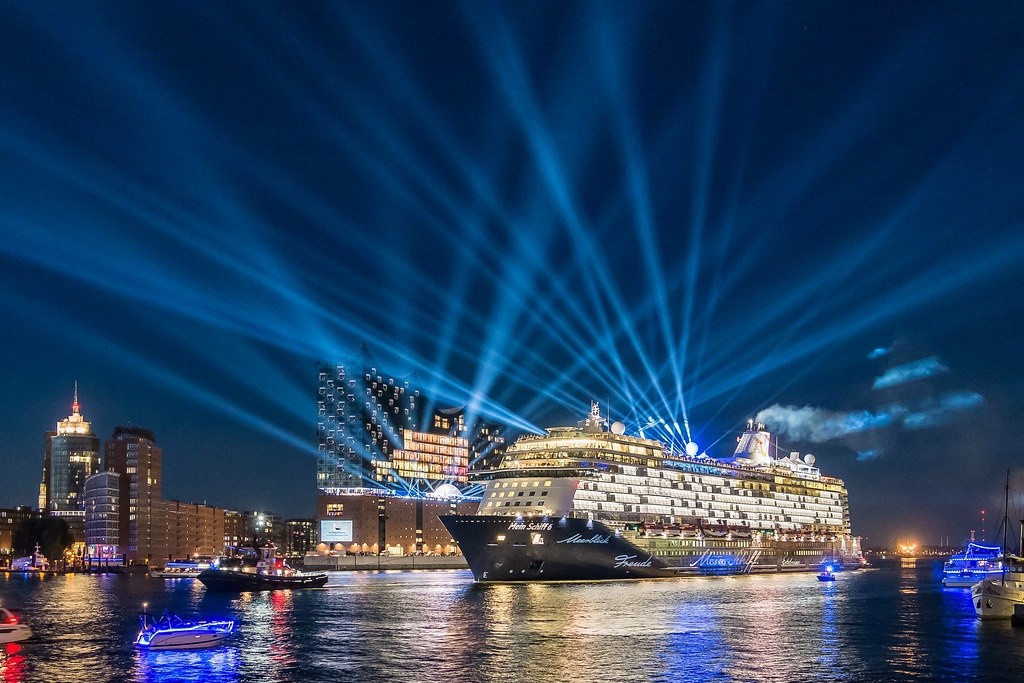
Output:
[783,530,833,539]
[817,571,836,582]
[941,529,1017,587]
[148,557,212,578]
[0,607,34,645]
[730,525,751,538]
[664,524,680,536]
[132,602,234,650]
[702,524,729,537]
[196,552,329,591]
[638,520,664,536]
[682,526,697,537]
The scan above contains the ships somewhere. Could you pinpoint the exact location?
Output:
[434,400,874,586]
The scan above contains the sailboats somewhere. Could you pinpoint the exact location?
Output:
[968,465,1024,621]
